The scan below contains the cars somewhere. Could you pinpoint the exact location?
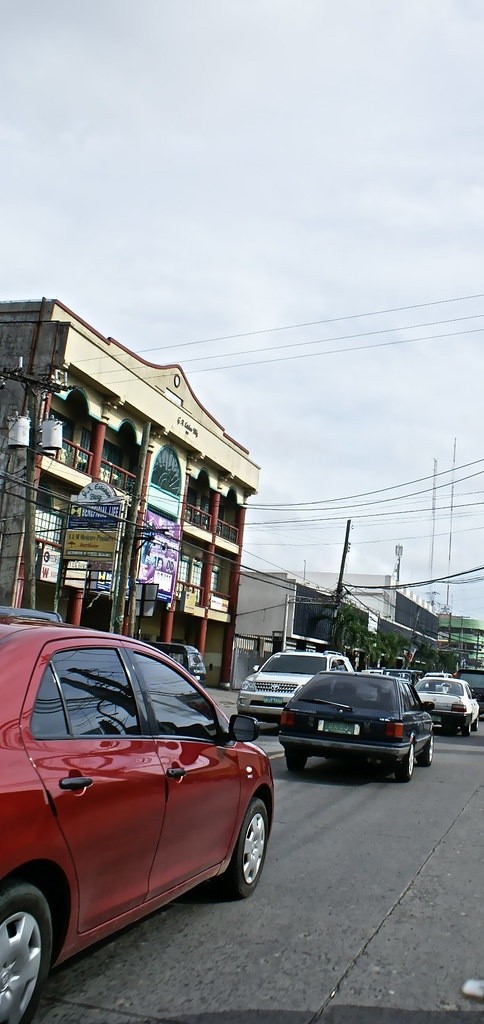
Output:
[0,615,277,1024]
[412,677,481,739]
[0,605,63,623]
[382,669,419,686]
[425,673,453,692]
[280,672,436,786]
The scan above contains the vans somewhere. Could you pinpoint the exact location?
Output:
[141,640,206,689]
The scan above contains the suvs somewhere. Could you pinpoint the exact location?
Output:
[453,667,484,716]
[239,648,352,723]
